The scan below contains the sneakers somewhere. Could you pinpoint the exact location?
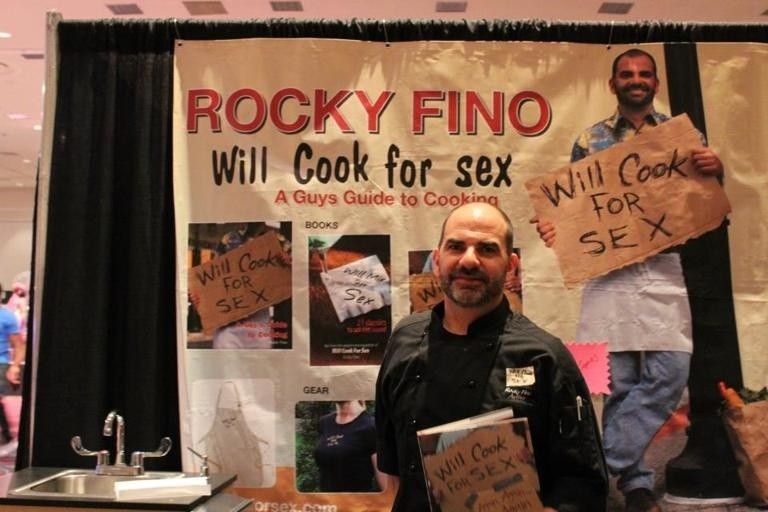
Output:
[626,488,660,511]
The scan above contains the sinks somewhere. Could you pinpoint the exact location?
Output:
[9,470,185,503]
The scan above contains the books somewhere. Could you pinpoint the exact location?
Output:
[417,407,547,512]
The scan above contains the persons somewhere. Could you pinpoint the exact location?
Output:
[189,232,291,348]
[0,304,23,444]
[528,47,723,512]
[315,400,387,493]
[372,201,610,512]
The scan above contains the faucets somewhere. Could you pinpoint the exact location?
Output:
[102,409,126,466]
